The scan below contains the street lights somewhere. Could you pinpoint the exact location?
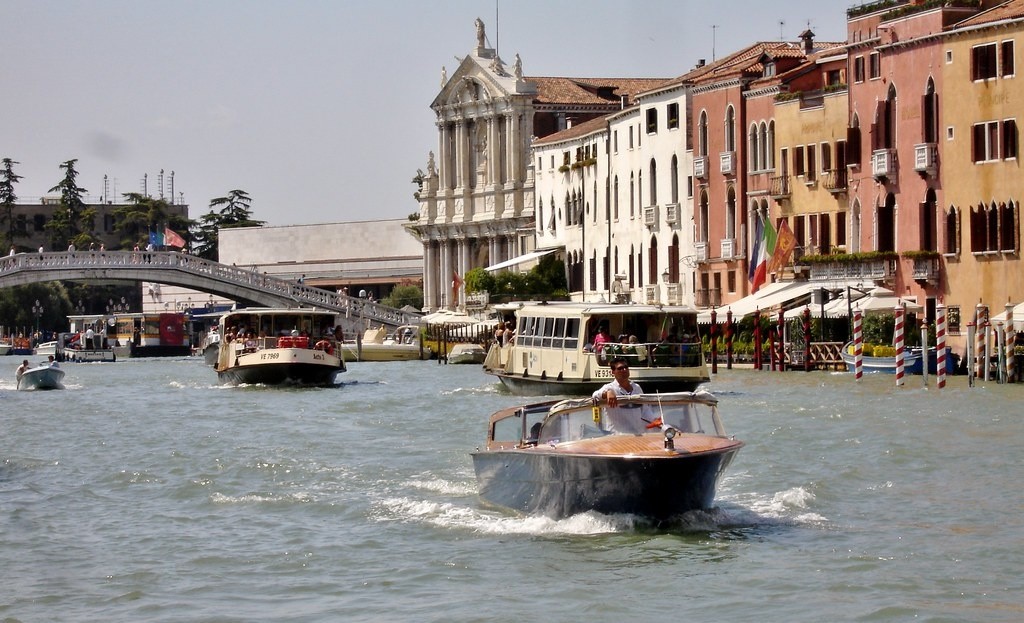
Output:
[177,297,194,312]
[32,300,42,340]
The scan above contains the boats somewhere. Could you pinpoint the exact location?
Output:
[842,338,955,376]
[213,302,348,387]
[11,339,34,355]
[447,343,487,364]
[65,313,195,357]
[17,366,65,392]
[341,325,432,361]
[56,332,115,362]
[201,324,221,365]
[468,388,744,523]
[0,345,12,355]
[483,296,712,395]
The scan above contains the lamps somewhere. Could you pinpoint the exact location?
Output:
[662,255,696,282]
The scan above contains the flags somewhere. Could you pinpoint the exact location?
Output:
[453,269,463,308]
[147,228,186,248]
[748,210,796,295]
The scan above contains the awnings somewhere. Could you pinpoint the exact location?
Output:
[697,282,821,324]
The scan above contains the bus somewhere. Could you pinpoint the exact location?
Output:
[184,306,233,347]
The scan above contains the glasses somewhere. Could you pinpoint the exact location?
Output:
[613,365,628,370]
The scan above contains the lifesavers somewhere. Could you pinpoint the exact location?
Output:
[5,338,8,343]
[313,340,334,355]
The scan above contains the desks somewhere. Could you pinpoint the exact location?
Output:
[245,347,264,353]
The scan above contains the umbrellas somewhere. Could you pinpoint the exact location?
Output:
[769,286,923,326]
[989,301,1024,333]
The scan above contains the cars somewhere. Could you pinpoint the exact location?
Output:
[39,340,58,348]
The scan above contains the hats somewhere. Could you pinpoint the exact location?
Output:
[344,287,348,289]
[618,334,627,340]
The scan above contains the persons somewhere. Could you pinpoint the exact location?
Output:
[489,56,506,77]
[440,65,447,89]
[224,262,376,353]
[39,244,44,260]
[475,17,486,45]
[426,150,435,174]
[15,327,108,390]
[526,422,542,445]
[10,246,15,263]
[379,324,415,344]
[493,320,693,366]
[511,53,522,81]
[592,357,655,436]
[68,241,188,264]
